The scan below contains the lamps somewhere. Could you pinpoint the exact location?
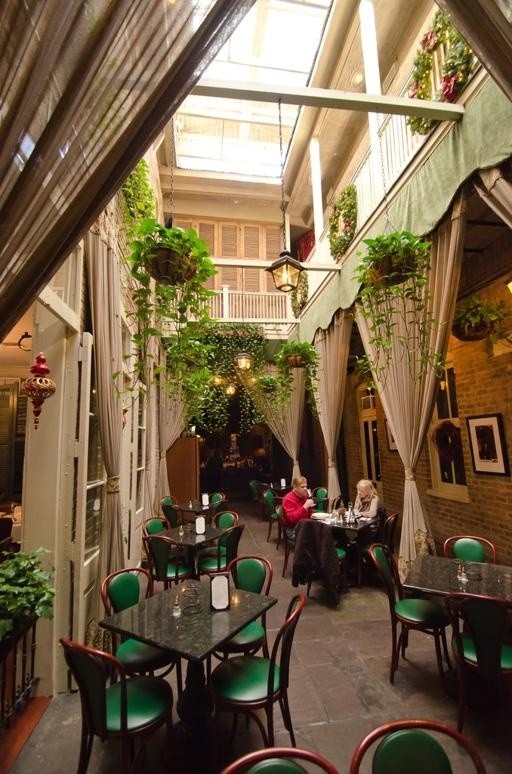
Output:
[226,382,237,393]
[265,99,307,292]
[234,266,254,371]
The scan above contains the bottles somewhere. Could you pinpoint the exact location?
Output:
[189,500,192,509]
[456,563,468,584]
[270,483,273,488]
[311,502,362,527]
[225,456,254,468]
[179,525,184,537]
[173,596,181,617]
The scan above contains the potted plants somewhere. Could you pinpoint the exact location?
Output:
[273,341,322,420]
[351,232,452,397]
[452,295,511,342]
[112,217,218,415]
[255,376,284,422]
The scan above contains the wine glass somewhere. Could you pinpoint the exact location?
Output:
[11,502,20,521]
[307,489,316,507]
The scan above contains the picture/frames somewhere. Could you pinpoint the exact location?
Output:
[466,413,511,477]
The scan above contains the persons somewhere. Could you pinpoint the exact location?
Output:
[205,449,223,470]
[281,475,317,541]
[336,478,381,588]
